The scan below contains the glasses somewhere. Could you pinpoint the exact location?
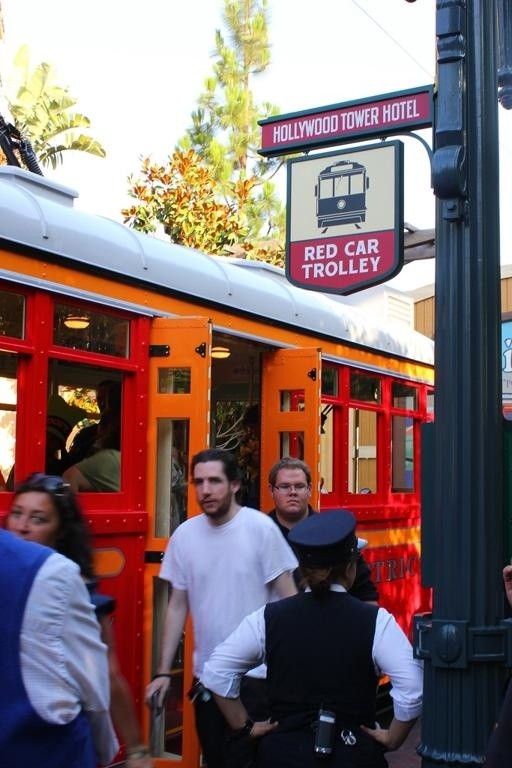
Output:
[273,483,309,493]
[24,473,70,496]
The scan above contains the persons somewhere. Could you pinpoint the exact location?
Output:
[9,476,152,768]
[145,449,300,768]
[46,379,189,537]
[0,530,120,768]
[485,566,512,768]
[200,508,424,768]
[239,405,260,510]
[266,458,379,605]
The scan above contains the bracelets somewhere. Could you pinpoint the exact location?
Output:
[126,746,150,758]
[233,719,254,739]
[152,674,174,680]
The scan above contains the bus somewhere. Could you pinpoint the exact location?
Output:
[0,164,436,768]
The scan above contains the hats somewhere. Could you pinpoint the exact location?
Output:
[288,511,367,570]
